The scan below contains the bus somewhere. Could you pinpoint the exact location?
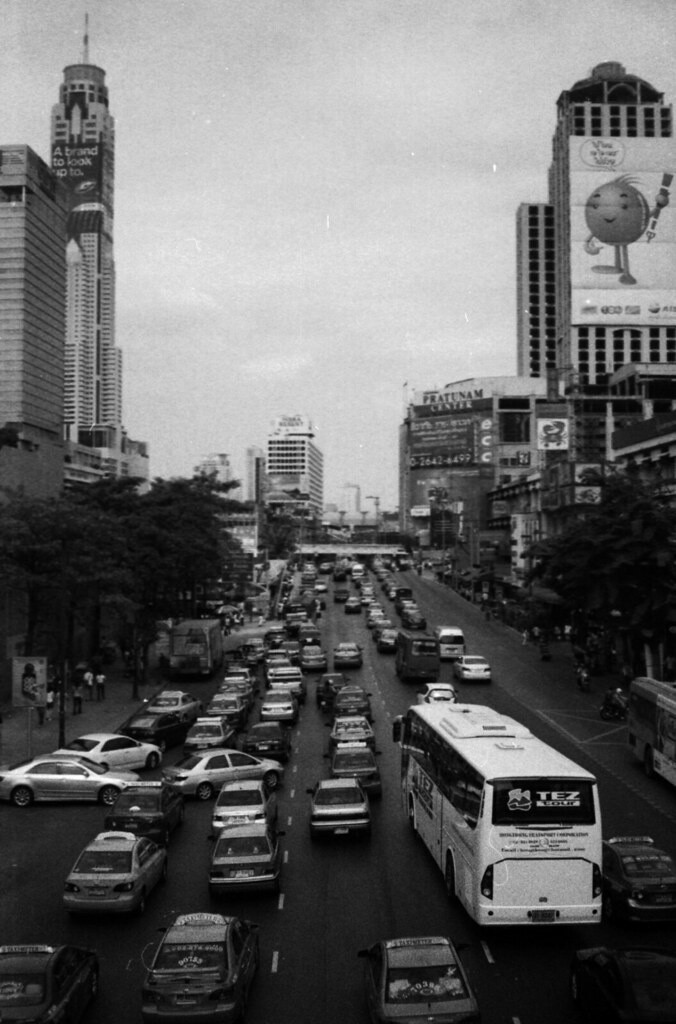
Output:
[167,619,226,680]
[625,676,676,792]
[391,701,604,929]
[393,630,442,684]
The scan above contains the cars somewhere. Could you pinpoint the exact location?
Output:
[183,717,237,757]
[416,682,459,704]
[314,673,350,707]
[242,722,294,763]
[0,754,144,808]
[306,777,372,840]
[159,748,284,802]
[218,553,451,701]
[205,692,252,723]
[145,691,205,723]
[207,822,286,898]
[137,912,260,1024]
[331,685,373,723]
[333,642,365,669]
[358,935,483,1024]
[259,689,300,726]
[571,950,676,1024]
[452,655,492,683]
[322,741,384,804]
[62,831,168,916]
[603,836,676,927]
[118,713,195,753]
[104,781,187,840]
[51,732,164,772]
[0,945,100,1024]
[324,714,377,749]
[209,780,279,841]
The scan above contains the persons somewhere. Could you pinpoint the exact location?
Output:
[36,707,44,725]
[70,682,83,715]
[56,687,69,713]
[84,668,94,701]
[611,688,629,719]
[225,609,244,634]
[46,686,55,721]
[95,671,106,700]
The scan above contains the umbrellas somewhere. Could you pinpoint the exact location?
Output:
[218,605,238,614]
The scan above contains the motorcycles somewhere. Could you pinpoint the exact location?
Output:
[599,698,629,722]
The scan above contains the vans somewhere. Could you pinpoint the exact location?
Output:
[432,624,466,660]
